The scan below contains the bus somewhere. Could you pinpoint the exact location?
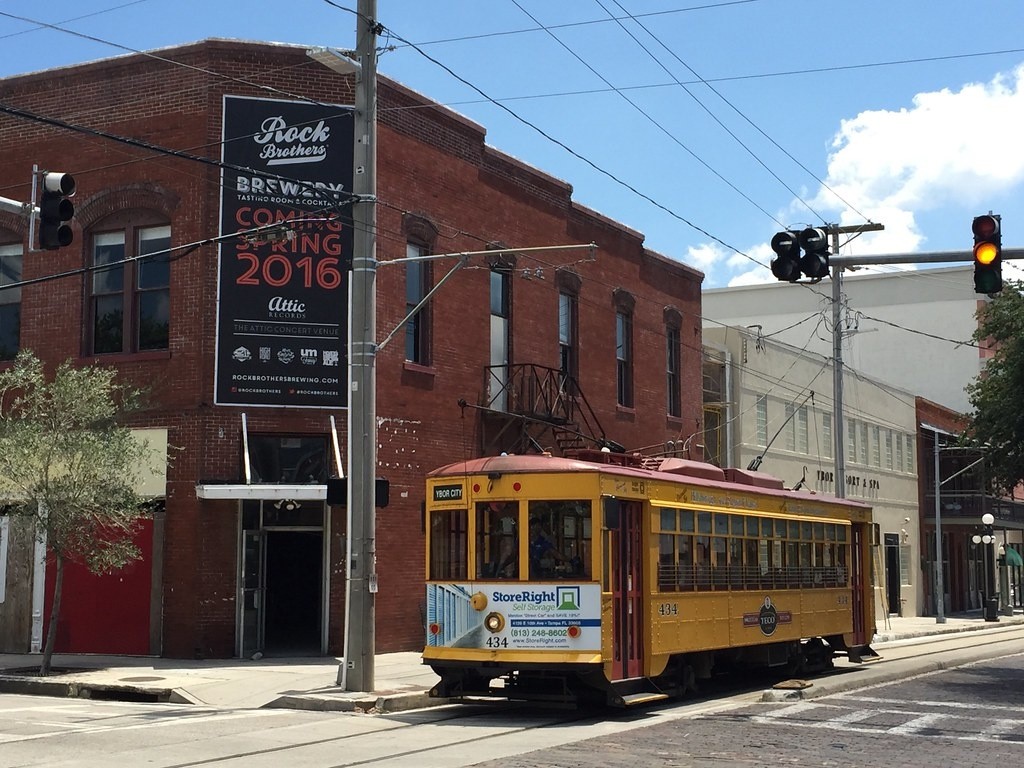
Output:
[420,438,879,708]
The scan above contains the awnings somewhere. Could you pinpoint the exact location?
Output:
[998,547,1024,567]
[1,428,169,517]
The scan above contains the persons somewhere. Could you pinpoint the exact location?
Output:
[495,514,583,579]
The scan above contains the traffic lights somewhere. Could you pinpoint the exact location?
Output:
[38,172,77,250]
[771,229,801,281]
[797,226,829,278]
[972,215,1003,293]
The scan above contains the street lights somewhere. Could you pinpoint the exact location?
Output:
[971,513,1000,622]
